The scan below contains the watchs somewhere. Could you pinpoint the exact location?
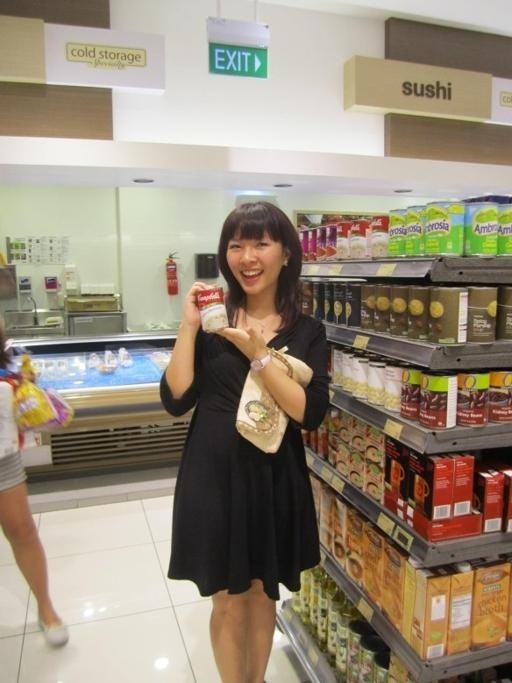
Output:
[246,349,273,371]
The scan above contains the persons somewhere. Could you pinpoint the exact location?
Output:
[0,325,71,647]
[160,201,329,683]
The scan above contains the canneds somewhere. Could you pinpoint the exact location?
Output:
[430,287,468,346]
[405,206,426,255]
[465,202,499,258]
[401,367,421,421]
[298,216,389,260]
[490,371,511,424]
[302,282,362,328]
[418,371,457,433]
[330,347,403,416]
[390,209,405,256]
[494,204,512,257]
[409,288,431,342]
[360,284,373,329]
[371,285,390,333]
[457,373,490,429]
[500,286,512,341]
[391,286,408,334]
[345,621,389,682]
[469,287,498,344]
[197,288,230,332]
[425,202,465,257]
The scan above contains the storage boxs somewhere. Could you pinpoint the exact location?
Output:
[65,292,122,312]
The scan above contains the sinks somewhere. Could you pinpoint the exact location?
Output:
[7,324,61,335]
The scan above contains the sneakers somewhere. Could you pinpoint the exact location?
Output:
[36,616,68,646]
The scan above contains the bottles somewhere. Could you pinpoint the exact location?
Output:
[291,559,359,681]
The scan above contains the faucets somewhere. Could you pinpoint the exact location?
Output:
[27,296,39,325]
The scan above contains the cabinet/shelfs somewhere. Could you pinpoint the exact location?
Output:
[64,310,127,336]
[274,254,511,683]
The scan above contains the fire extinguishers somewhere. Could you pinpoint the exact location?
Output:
[166,252,179,295]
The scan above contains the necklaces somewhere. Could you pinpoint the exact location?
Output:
[242,311,280,336]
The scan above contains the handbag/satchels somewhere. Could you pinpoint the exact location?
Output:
[235,346,313,455]
